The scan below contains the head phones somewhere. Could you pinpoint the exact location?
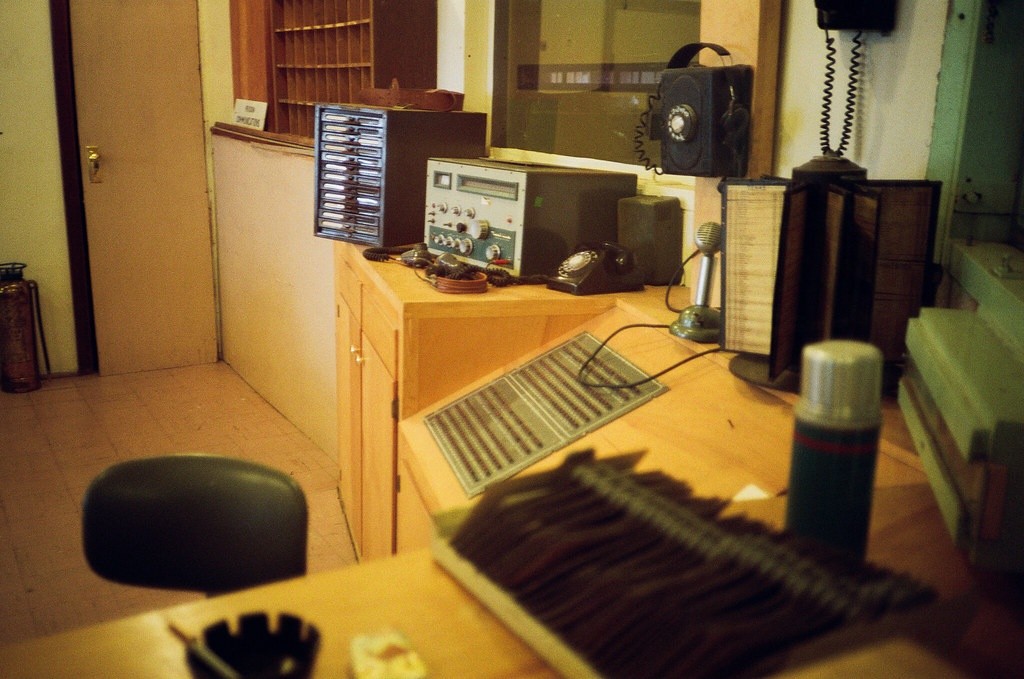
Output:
[645,43,750,146]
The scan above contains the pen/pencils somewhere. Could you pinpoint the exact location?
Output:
[166,619,240,679]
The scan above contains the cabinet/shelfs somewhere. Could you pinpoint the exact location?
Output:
[313,103,487,247]
[228,0,438,149]
[332,239,682,564]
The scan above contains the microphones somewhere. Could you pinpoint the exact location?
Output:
[669,221,722,343]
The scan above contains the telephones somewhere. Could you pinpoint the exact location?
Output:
[644,42,754,178]
[547,237,645,295]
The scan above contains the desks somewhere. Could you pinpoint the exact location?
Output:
[0,291,1024,679]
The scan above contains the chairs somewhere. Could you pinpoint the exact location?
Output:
[81,455,309,597]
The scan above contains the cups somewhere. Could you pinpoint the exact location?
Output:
[781,339,884,560]
